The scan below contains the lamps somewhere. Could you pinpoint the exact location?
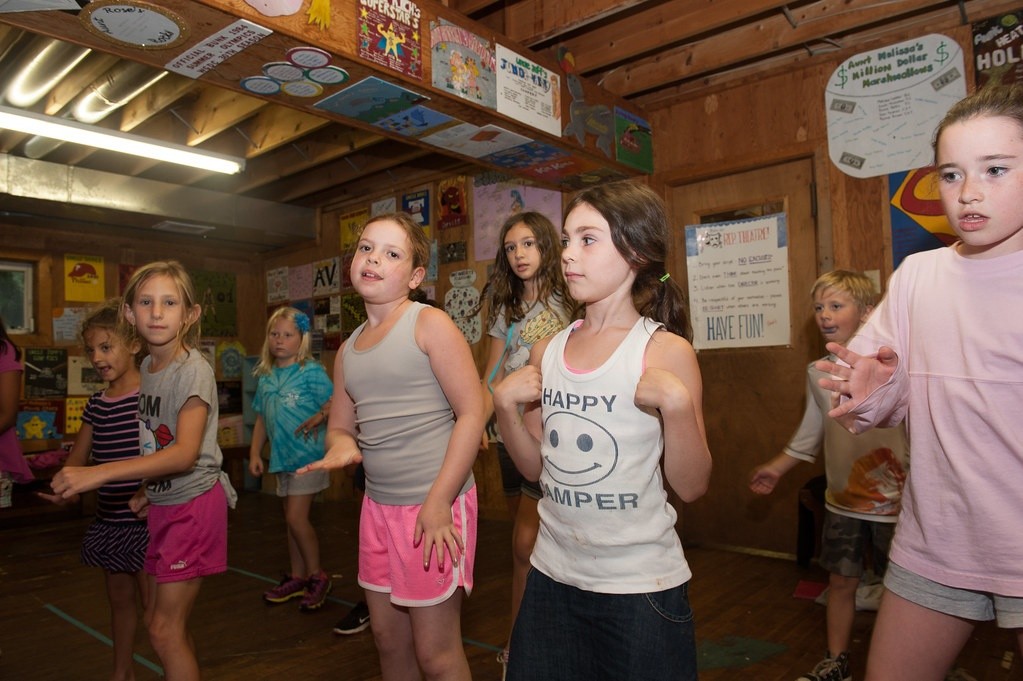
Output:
[0,103,246,176]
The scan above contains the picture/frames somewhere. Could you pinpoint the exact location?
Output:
[0,264,34,336]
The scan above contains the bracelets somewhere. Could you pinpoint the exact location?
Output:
[320,411,327,421]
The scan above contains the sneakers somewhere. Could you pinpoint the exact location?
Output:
[332,600,371,634]
[796,652,852,681]
[298,569,331,610]
[264,574,305,602]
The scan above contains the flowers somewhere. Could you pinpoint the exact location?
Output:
[294,312,311,333]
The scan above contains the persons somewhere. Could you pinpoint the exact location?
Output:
[38,298,151,681]
[751,268,907,681]
[479,211,573,664]
[494,179,713,681]
[51,260,237,681]
[297,213,486,680]
[0,317,35,484]
[815,81,1023,680]
[248,306,335,605]
